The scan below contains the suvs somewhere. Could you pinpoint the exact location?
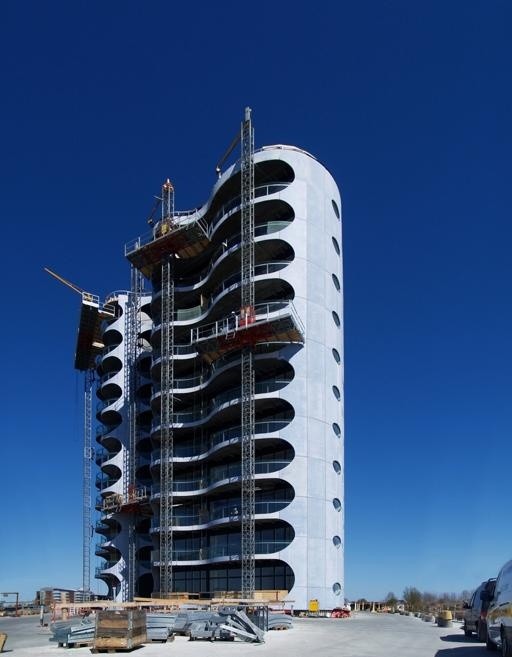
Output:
[479,556,512,656]
[461,577,496,640]
